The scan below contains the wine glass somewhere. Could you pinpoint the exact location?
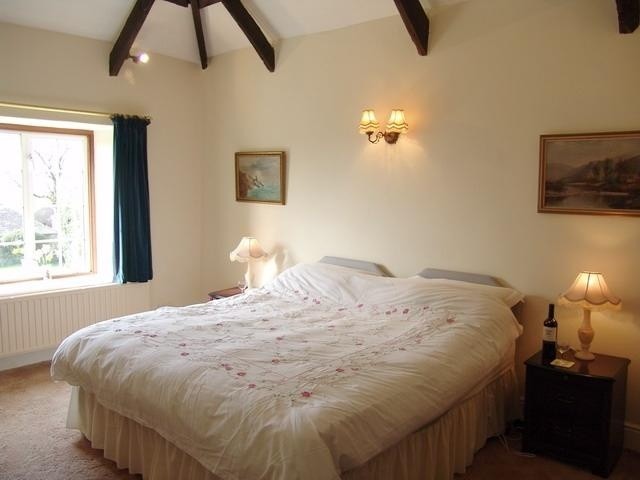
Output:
[238,279,247,293]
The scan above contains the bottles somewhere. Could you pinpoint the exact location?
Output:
[541,303,558,365]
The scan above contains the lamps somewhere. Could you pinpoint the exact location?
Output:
[357,108,409,144]
[559,269,623,361]
[229,235,271,293]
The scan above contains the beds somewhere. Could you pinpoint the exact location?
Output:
[62,254,526,480]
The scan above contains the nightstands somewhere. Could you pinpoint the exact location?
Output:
[524,344,631,480]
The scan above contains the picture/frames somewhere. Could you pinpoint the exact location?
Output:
[537,130,640,217]
[233,150,286,206]
